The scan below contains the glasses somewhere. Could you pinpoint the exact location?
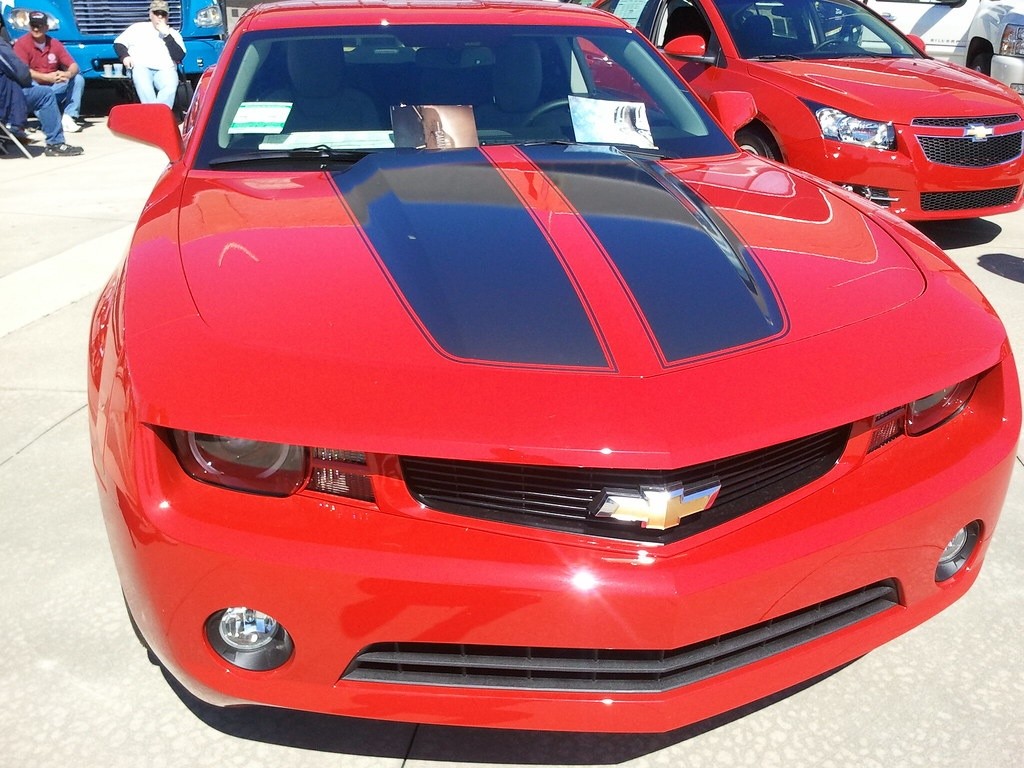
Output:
[30,22,45,28]
[154,11,167,17]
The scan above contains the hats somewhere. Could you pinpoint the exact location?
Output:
[29,11,47,26]
[150,0,170,14]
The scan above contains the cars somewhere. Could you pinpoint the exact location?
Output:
[87,0,1023,734]
[751,1,1023,97]
[574,0,1024,217]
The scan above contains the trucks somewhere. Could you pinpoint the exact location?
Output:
[0,2,226,104]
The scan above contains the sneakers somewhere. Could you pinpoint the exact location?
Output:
[61,118,82,133]
[7,132,33,146]
[44,143,83,156]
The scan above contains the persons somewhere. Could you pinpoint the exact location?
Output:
[112,0,187,111]
[14,11,84,133]
[0,14,83,157]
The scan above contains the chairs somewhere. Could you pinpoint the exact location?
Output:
[0,119,34,159]
[734,14,773,58]
[266,36,380,129]
[126,59,190,122]
[665,8,714,58]
[474,39,562,128]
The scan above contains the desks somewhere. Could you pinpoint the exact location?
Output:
[101,74,129,82]
